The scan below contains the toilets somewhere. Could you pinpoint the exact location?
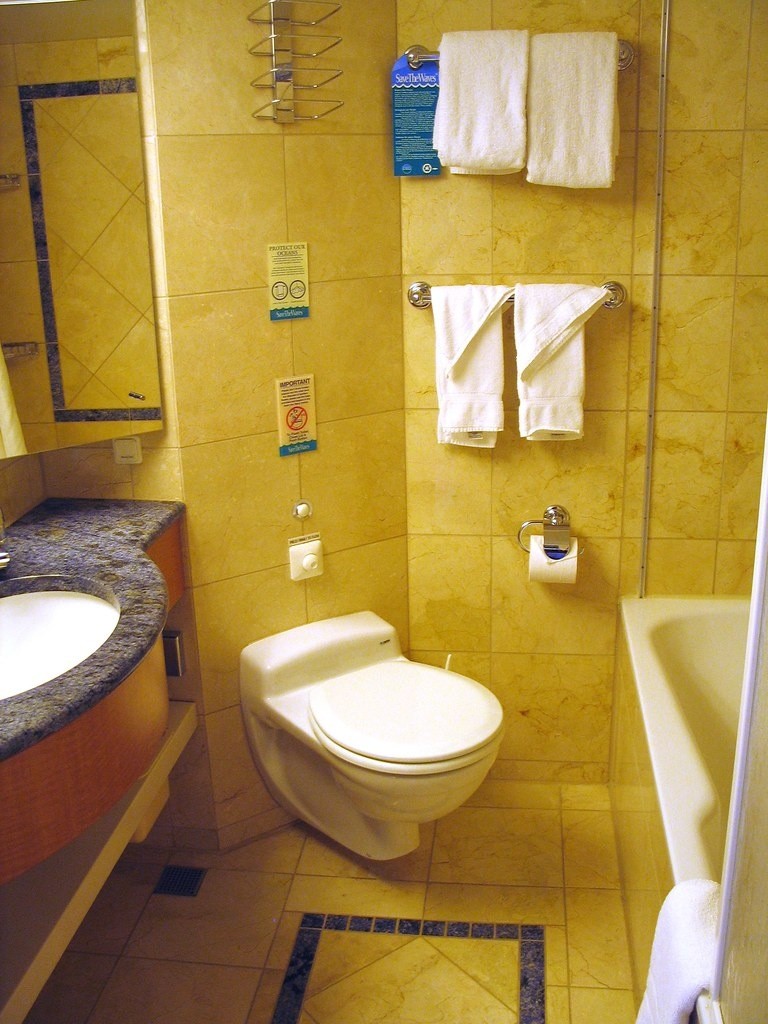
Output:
[237,608,508,864]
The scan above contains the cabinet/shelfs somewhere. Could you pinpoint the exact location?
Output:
[245,0,345,125]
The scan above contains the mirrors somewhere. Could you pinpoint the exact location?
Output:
[0,0,164,461]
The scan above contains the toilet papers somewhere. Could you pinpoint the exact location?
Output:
[526,532,579,587]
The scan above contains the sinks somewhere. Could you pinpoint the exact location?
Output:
[0,576,122,706]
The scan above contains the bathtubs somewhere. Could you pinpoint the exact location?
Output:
[606,588,768,1024]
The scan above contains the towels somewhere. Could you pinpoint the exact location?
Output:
[515,320,589,441]
[631,875,728,1024]
[431,306,509,451]
[527,31,624,189]
[428,280,514,377]
[512,283,618,387]
[430,27,533,190]
